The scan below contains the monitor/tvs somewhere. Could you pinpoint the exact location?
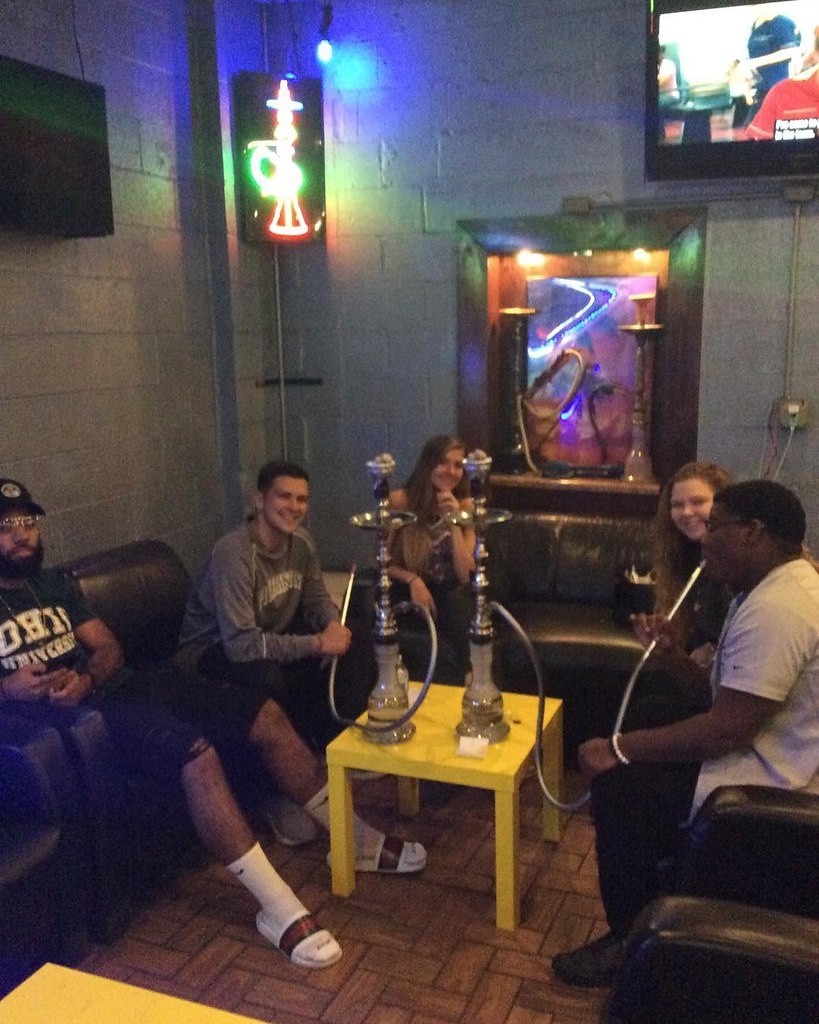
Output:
[0,55,114,239]
[643,0,819,185]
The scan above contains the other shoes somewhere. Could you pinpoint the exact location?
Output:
[551,930,627,986]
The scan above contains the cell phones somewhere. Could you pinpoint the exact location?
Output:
[44,649,80,675]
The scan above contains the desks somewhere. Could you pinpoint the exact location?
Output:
[1,963,265,1024]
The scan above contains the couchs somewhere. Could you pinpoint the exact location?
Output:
[478,513,648,667]
[602,787,819,1024]
[0,540,238,999]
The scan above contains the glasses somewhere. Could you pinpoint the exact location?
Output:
[704,517,752,533]
[0,515,44,536]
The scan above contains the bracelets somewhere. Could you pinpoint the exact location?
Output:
[407,573,417,585]
[611,732,630,765]
[317,632,324,654]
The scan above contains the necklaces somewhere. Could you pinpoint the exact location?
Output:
[0,576,47,653]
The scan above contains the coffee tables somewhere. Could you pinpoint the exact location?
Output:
[325,680,563,930]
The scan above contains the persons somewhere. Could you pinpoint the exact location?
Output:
[552,480,819,987]
[378,434,482,687]
[175,461,397,848]
[0,478,429,971]
[657,0,819,141]
[648,460,819,681]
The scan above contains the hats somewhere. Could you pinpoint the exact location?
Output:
[0,478,46,515]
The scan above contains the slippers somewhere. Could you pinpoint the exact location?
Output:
[255,910,344,968]
[325,835,427,873]
